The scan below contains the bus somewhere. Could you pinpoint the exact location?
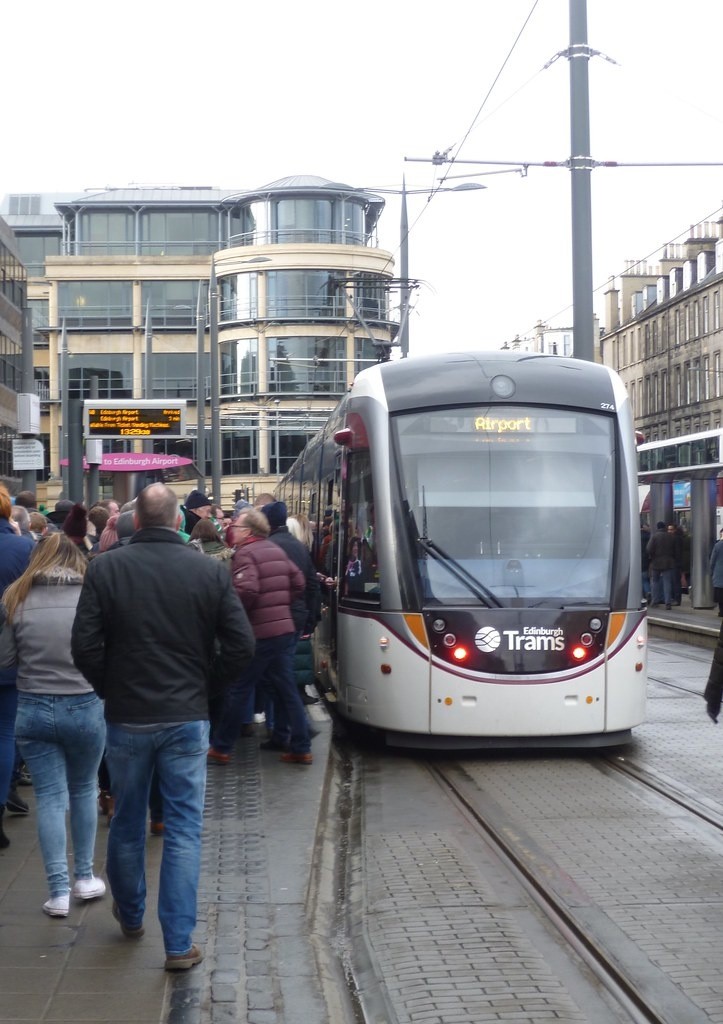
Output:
[632,426,723,606]
[267,349,650,753]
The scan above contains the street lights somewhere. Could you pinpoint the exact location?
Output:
[141,297,192,453]
[208,251,273,507]
[397,173,487,357]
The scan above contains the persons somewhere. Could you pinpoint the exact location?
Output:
[72,482,258,971]
[640,517,691,610]
[0,483,323,918]
[708,528,723,617]
[703,617,723,725]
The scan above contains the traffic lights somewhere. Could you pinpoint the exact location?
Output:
[232,489,241,503]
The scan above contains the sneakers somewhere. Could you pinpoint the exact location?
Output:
[43,896,70,916]
[73,874,106,899]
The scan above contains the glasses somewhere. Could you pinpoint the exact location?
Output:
[229,523,244,529]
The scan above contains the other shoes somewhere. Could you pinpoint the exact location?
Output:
[281,754,313,765]
[665,604,672,610]
[15,764,33,785]
[98,790,109,814]
[164,945,203,968]
[300,691,320,705]
[106,797,114,824]
[149,821,163,833]
[261,736,289,751]
[112,899,146,938]
[207,747,230,763]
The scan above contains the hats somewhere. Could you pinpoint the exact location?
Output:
[64,504,88,538]
[186,489,211,509]
[260,502,287,527]
[98,515,119,552]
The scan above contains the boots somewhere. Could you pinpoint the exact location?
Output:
[5,772,31,811]
[0,803,9,847]
